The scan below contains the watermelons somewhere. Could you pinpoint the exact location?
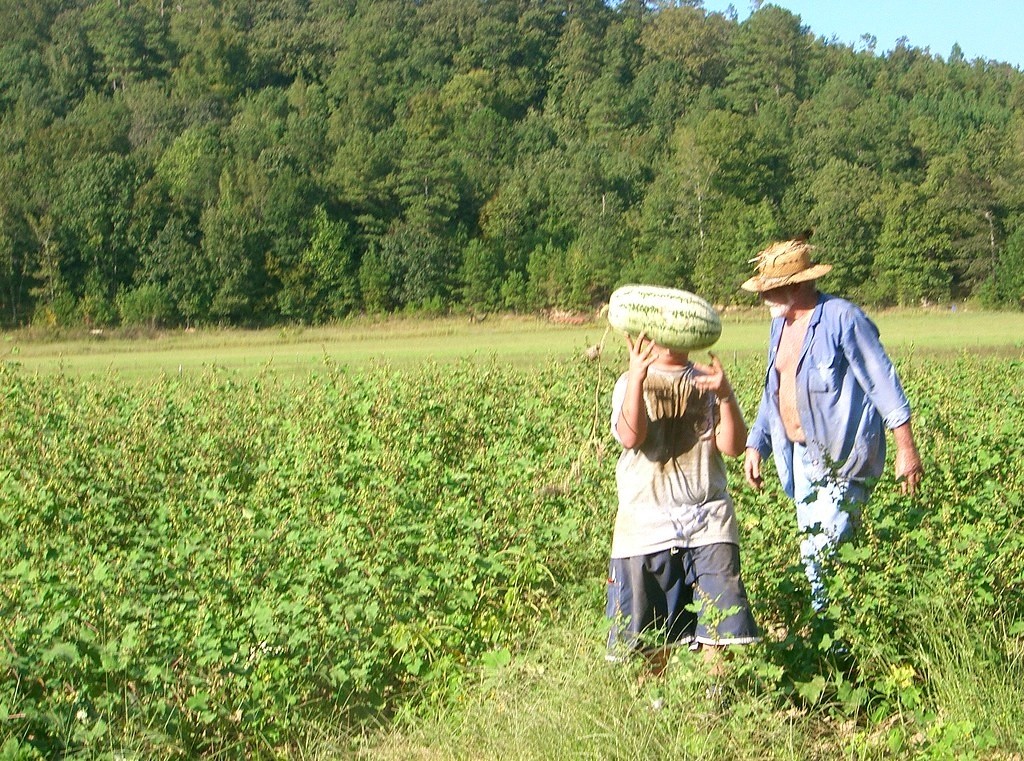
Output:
[608,286,722,350]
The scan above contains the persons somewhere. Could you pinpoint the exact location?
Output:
[744,239,924,652]
[604,328,764,671]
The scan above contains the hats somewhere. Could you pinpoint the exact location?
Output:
[741,239,832,293]
